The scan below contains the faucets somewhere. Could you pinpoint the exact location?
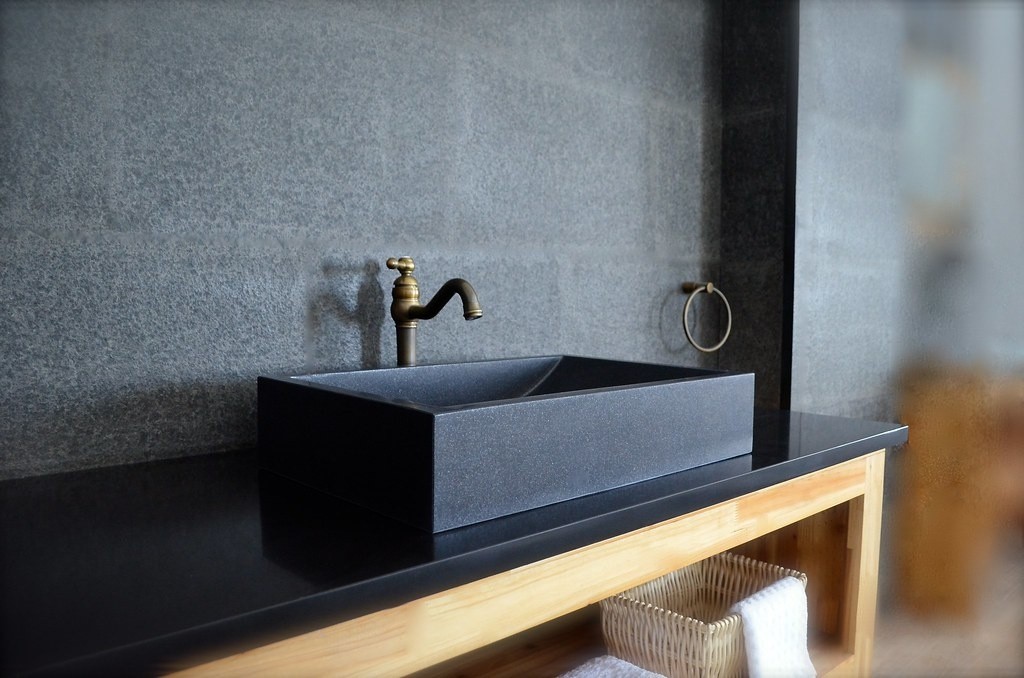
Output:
[386,256,484,368]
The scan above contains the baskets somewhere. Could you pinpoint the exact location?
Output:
[599,551,808,678]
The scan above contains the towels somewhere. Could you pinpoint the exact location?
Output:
[723,576,818,678]
[561,654,668,678]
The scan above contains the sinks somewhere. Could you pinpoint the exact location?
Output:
[255,354,753,537]
